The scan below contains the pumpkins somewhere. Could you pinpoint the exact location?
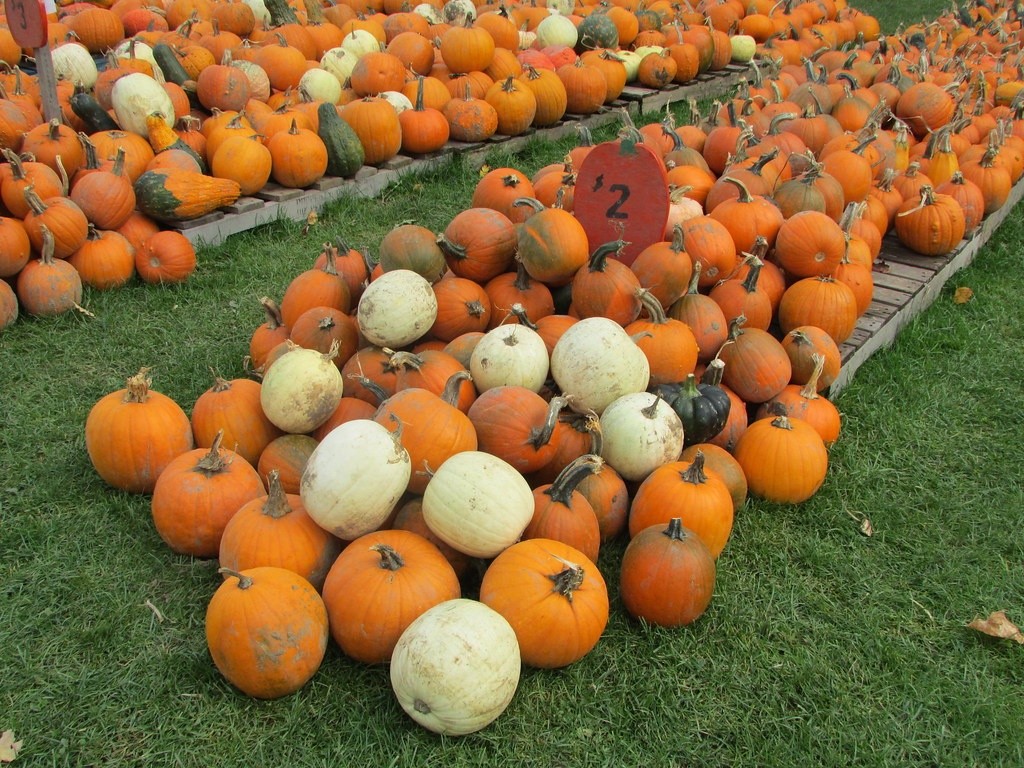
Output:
[0,1,1024,737]
